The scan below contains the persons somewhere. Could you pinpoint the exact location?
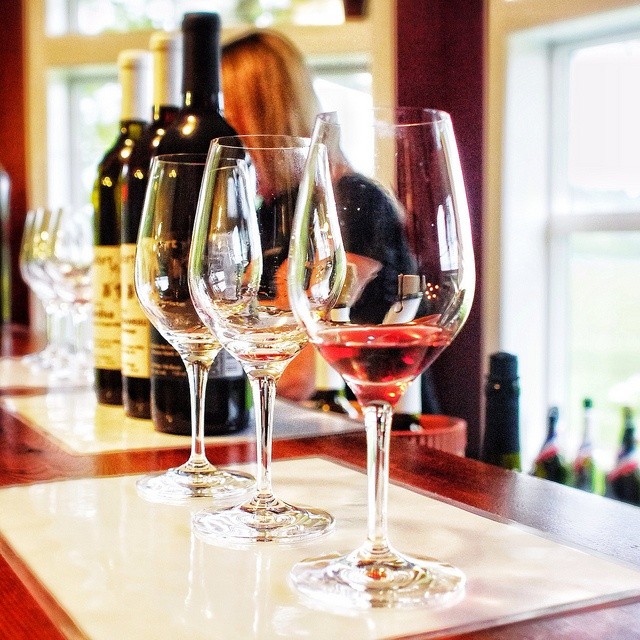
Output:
[216,28,418,416]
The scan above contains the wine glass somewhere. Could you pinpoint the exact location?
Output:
[288,102,478,617]
[52,209,98,382]
[187,135,348,545]
[29,209,53,380]
[17,208,30,378]
[134,153,260,512]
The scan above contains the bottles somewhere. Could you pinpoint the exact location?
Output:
[94,50,150,406]
[119,33,174,418]
[563,398,606,497]
[481,350,521,474]
[604,405,640,507]
[528,405,570,482]
[150,11,249,440]
